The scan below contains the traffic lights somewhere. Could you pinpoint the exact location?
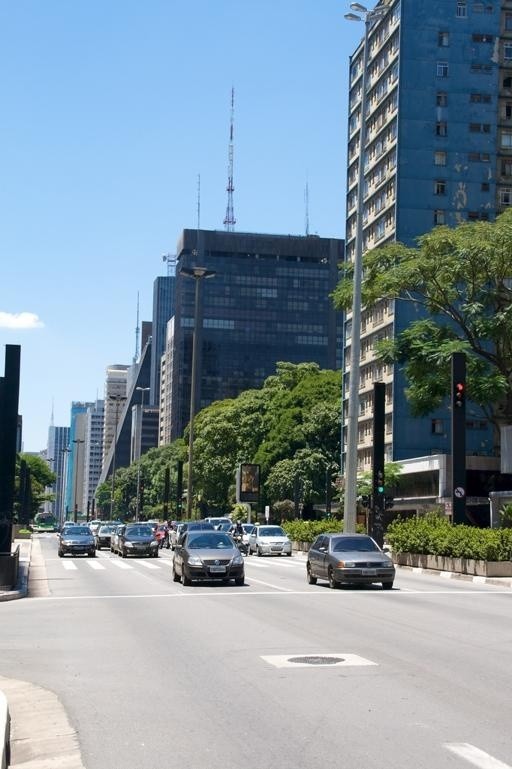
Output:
[455,383,464,408]
[378,471,384,494]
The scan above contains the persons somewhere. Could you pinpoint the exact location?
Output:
[232,521,247,536]
[169,526,176,550]
[164,526,170,548]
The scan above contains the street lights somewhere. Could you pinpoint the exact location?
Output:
[178,267,215,518]
[45,387,151,520]
[344,3,391,535]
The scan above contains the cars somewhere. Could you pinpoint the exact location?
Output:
[58,520,169,558]
[170,517,292,587]
[307,533,396,589]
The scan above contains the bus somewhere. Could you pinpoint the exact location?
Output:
[35,512,55,532]
